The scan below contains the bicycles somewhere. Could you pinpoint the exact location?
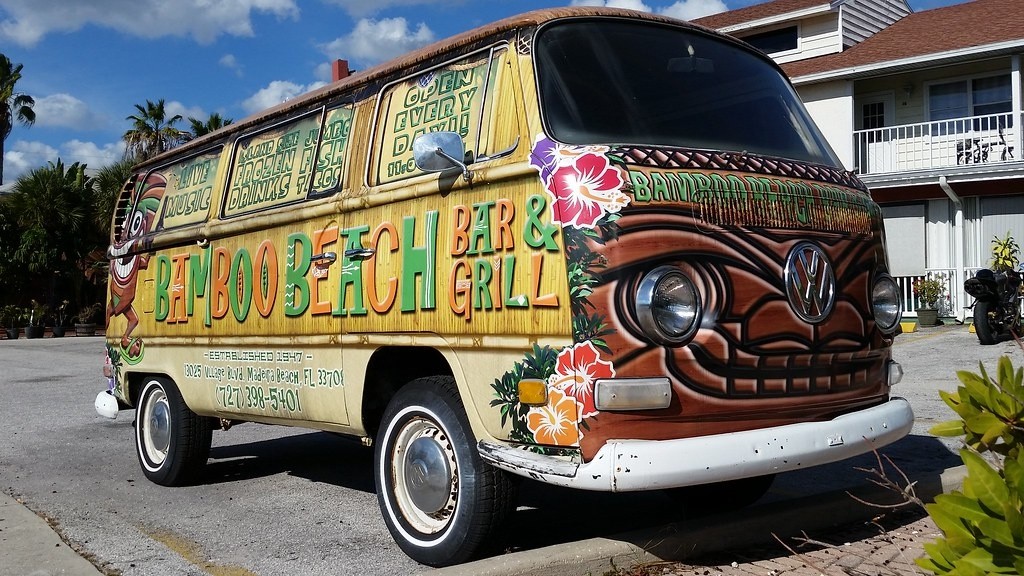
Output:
[957,122,1014,164]
[1002,157,1006,158]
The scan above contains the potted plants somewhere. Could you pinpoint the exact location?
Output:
[47,300,70,338]
[23,300,49,339]
[70,303,103,337]
[0,304,22,340]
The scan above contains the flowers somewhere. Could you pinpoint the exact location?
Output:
[912,272,951,308]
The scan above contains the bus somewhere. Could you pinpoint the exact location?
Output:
[95,5,915,566]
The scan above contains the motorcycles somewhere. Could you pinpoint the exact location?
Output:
[963,267,1022,345]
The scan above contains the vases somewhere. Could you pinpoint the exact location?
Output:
[915,309,939,327]
[896,322,916,333]
